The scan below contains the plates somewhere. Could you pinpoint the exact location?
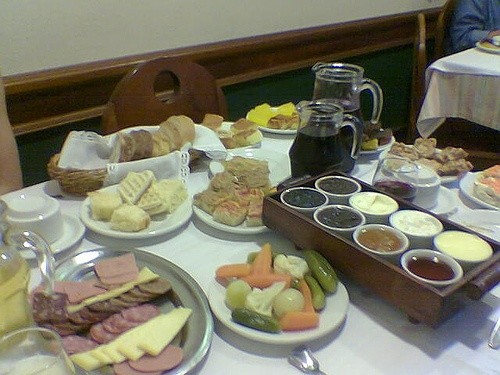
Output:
[358,128,395,154]
[34,247,214,375]
[194,121,262,154]
[399,246,463,286]
[193,180,280,234]
[2,210,86,257]
[432,230,494,272]
[449,209,500,245]
[281,186,328,220]
[313,205,366,239]
[79,184,193,239]
[475,42,500,54]
[421,186,458,217]
[316,174,362,204]
[378,145,466,187]
[207,244,349,344]
[208,148,291,188]
[389,208,443,251]
[349,191,398,226]
[352,223,409,266]
[246,107,299,134]
[459,171,500,210]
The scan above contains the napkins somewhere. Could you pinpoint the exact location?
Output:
[190,124,227,161]
[103,150,190,188]
[57,130,121,170]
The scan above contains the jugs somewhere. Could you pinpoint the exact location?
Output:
[311,61,383,126]
[0,199,56,351]
[288,102,363,178]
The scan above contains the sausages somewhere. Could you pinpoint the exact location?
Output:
[32,253,184,375]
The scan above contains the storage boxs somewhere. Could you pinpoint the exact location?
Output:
[263,169,500,328]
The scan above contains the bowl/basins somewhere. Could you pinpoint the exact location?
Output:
[393,163,441,210]
[4,194,63,245]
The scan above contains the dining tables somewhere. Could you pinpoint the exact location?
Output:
[416,47,500,139]
[0,122,500,375]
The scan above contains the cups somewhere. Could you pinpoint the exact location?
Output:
[371,156,418,204]
[0,327,75,375]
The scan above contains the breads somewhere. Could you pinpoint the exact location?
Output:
[266,112,298,130]
[360,120,393,150]
[111,114,196,163]
[202,113,263,149]
[224,155,270,186]
[88,169,188,232]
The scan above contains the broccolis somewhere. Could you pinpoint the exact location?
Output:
[245,281,286,317]
[274,253,309,280]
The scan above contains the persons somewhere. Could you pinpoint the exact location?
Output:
[445,0,500,56]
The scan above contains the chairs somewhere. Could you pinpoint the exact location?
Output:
[102,55,226,136]
[408,13,500,173]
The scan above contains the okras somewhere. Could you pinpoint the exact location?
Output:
[306,275,325,309]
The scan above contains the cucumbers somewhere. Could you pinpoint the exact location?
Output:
[305,249,338,293]
